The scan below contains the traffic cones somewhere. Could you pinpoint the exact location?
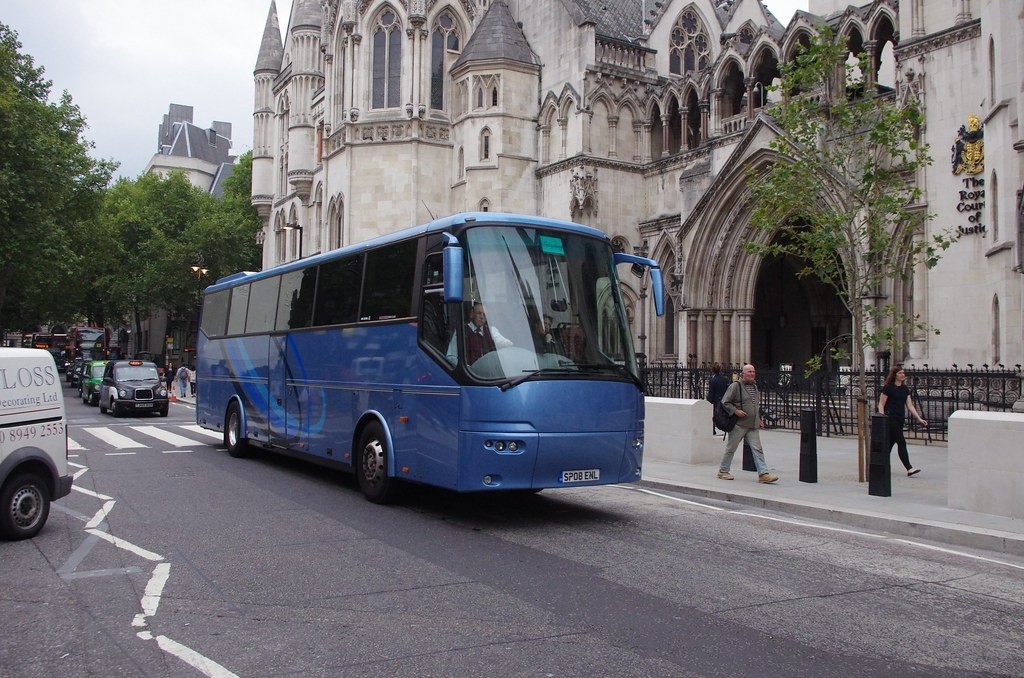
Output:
[170,386,178,401]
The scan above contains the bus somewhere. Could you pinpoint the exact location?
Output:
[191,209,666,505]
[20,327,107,370]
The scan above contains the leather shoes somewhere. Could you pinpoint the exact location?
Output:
[718,468,733,480]
[758,473,778,484]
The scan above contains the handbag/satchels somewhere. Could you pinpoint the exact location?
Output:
[713,398,740,433]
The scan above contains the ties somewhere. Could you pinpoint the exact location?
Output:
[475,327,481,337]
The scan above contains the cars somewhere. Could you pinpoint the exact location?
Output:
[66,360,115,403]
[98,360,170,420]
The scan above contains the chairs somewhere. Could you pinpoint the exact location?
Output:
[425,292,539,355]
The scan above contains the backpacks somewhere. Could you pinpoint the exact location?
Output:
[179,366,188,381]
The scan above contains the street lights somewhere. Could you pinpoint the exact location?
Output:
[190,260,211,336]
[283,223,303,260]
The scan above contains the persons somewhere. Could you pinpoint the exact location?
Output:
[175,362,190,398]
[446,303,515,370]
[716,363,779,484]
[707,363,730,435]
[188,365,196,397]
[878,364,929,477]
[164,362,176,393]
[525,314,563,355]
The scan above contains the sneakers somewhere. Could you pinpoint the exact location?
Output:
[167,390,197,398]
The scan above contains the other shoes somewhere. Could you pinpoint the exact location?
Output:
[908,469,921,476]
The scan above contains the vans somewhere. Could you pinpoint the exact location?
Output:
[0,346,75,541]
